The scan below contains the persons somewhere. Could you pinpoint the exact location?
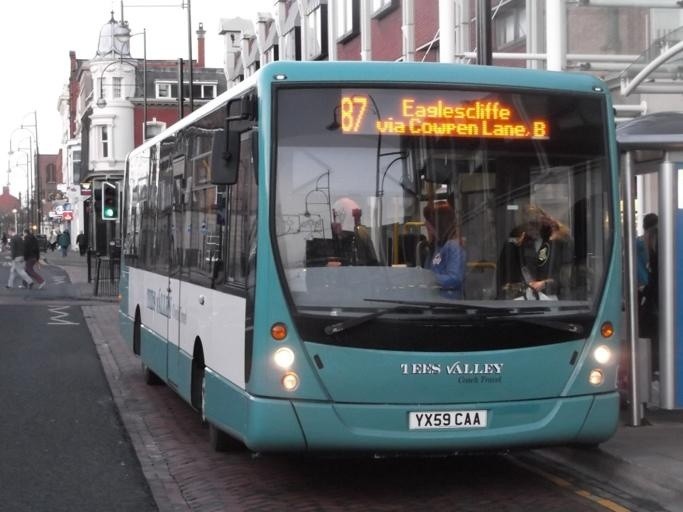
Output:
[495,204,562,301]
[636,212,659,285]
[0,229,86,290]
[425,199,468,302]
[305,194,379,269]
[638,224,659,338]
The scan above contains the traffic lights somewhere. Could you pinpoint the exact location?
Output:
[104,184,118,220]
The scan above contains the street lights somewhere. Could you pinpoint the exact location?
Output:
[98,59,147,141]
[6,111,42,237]
[117,1,193,111]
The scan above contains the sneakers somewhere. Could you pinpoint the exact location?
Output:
[6,280,46,289]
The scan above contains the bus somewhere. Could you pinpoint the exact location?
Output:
[103,58,624,456]
